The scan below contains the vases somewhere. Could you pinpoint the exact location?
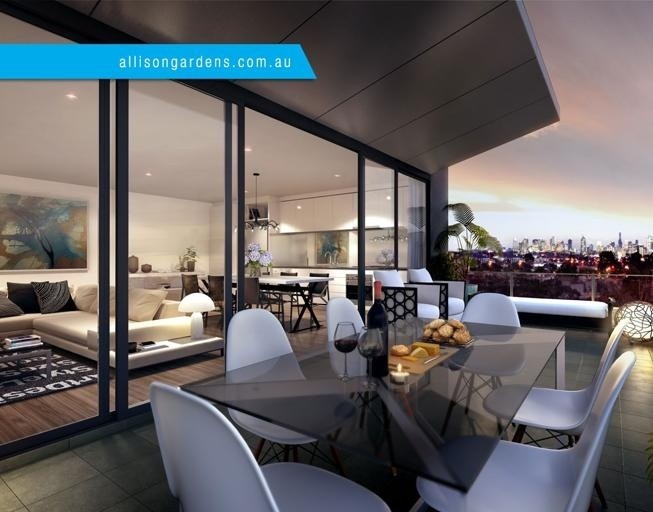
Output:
[248,261,260,277]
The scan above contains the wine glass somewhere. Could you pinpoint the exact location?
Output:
[332,321,356,383]
[356,325,382,393]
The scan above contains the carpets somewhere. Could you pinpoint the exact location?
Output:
[0,346,114,405]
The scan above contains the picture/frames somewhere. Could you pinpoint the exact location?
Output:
[0,191,90,274]
[314,231,350,268]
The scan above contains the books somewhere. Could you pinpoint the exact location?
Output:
[5,334,42,349]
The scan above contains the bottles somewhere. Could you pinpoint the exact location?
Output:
[366,280,391,376]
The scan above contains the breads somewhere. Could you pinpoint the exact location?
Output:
[392,342,439,363]
[424,316,470,342]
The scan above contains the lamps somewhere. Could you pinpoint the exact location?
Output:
[368,229,406,243]
[231,172,280,233]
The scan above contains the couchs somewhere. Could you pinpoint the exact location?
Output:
[0,275,223,378]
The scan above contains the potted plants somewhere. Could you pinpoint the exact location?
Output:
[182,246,198,271]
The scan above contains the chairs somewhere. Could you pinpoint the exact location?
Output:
[146,267,636,512]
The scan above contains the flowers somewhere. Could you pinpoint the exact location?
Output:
[242,241,273,267]
[375,248,399,266]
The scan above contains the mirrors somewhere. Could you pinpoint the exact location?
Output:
[268,226,407,269]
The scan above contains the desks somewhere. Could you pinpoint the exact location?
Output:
[128,270,203,292]
[108,334,224,377]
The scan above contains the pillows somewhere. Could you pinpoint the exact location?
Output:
[0,279,168,324]
